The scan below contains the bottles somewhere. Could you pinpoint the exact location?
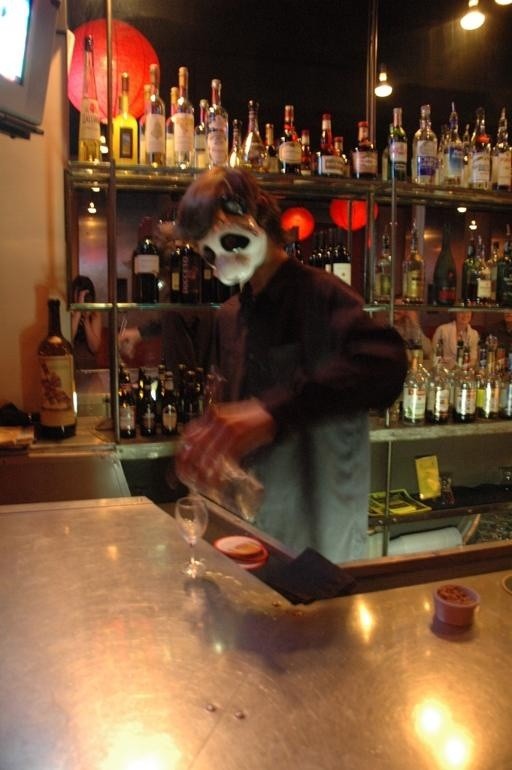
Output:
[35,298,77,439]
[76,30,511,189]
[101,335,511,437]
[109,190,512,304]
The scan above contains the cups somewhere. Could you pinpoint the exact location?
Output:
[174,422,262,523]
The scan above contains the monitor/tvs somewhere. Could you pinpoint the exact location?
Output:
[0,0,57,126]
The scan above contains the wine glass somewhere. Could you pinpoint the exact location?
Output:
[175,499,209,578]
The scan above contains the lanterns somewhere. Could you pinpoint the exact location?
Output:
[328,193,378,232]
[66,19,161,124]
[280,206,315,243]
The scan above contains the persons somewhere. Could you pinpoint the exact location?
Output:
[434,312,479,359]
[389,296,432,359]
[483,312,511,359]
[119,221,212,384]
[173,168,407,563]
[67,275,102,373]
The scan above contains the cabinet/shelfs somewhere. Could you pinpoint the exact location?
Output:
[66,164,512,559]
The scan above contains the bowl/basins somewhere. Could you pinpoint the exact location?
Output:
[433,585,479,626]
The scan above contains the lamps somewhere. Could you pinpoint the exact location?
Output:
[458,0,483,31]
[375,66,391,98]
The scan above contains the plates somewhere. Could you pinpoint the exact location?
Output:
[214,535,270,563]
